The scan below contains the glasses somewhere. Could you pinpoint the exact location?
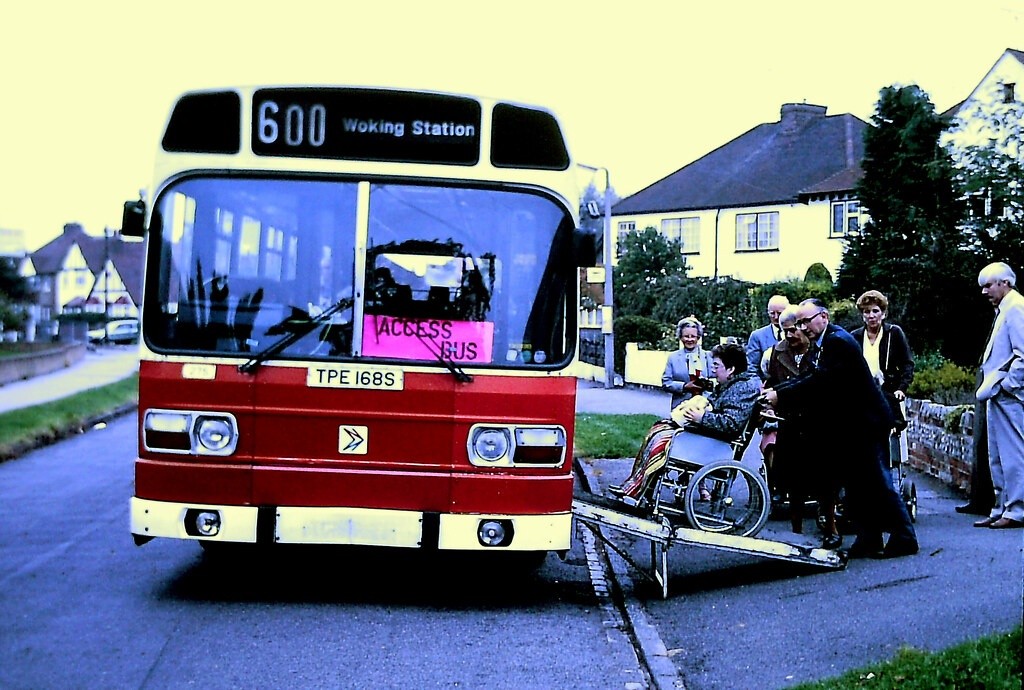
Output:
[795,312,821,329]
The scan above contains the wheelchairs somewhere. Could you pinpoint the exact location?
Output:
[621,386,920,540]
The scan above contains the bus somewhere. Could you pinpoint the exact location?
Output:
[113,79,614,588]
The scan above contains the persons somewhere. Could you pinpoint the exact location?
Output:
[607,262,1024,559]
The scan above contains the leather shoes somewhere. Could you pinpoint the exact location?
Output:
[822,531,840,548]
[840,537,885,559]
[952,500,987,512]
[874,541,920,559]
[971,513,1023,529]
[609,483,626,497]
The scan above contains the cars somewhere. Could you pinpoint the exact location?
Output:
[88,316,140,345]
